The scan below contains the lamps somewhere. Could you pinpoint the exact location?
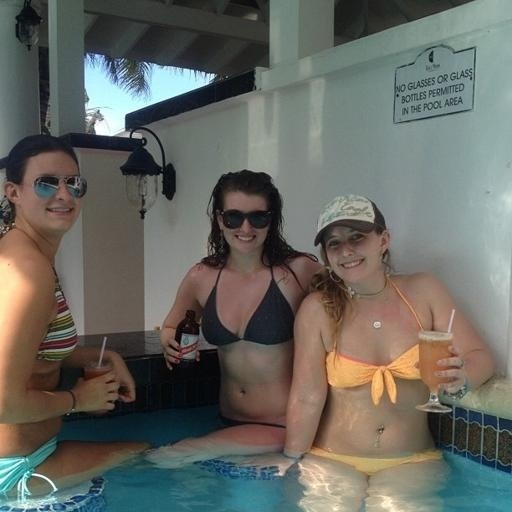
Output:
[15,0,43,54]
[119,127,176,219]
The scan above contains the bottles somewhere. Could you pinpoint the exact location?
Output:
[174,310,199,369]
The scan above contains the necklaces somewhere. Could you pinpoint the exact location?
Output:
[349,278,389,298]
[366,317,383,329]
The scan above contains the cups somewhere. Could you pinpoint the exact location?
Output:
[82,363,114,415]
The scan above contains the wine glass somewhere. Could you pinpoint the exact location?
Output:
[414,331,453,413]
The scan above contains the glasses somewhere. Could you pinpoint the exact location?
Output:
[33,175,87,198]
[217,209,272,228]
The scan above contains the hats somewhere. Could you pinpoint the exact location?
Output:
[314,194,386,247]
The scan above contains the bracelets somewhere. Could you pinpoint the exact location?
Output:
[281,451,304,461]
[439,378,468,400]
[68,388,76,416]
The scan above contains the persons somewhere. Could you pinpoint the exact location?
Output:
[1,132,149,499]
[137,167,324,481]
[239,191,498,511]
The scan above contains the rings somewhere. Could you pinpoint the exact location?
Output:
[460,359,465,368]
